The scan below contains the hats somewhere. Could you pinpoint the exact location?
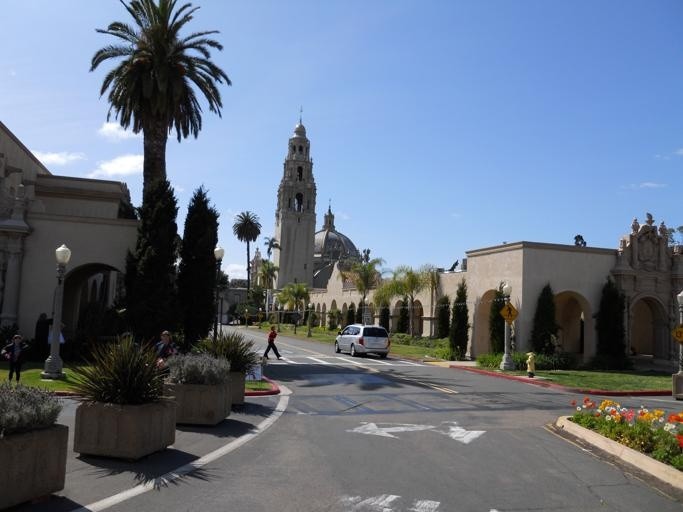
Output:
[160,330,170,336]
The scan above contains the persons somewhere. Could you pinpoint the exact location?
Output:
[114,331,140,351]
[150,329,180,377]
[29,312,54,362]
[262,324,283,361]
[0,334,30,385]
[524,349,536,379]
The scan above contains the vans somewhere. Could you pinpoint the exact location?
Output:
[334,322,391,359]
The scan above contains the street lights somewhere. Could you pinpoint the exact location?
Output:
[500,280,515,371]
[40,242,71,380]
[212,245,224,357]
[670,290,683,400]
[243,301,313,338]
